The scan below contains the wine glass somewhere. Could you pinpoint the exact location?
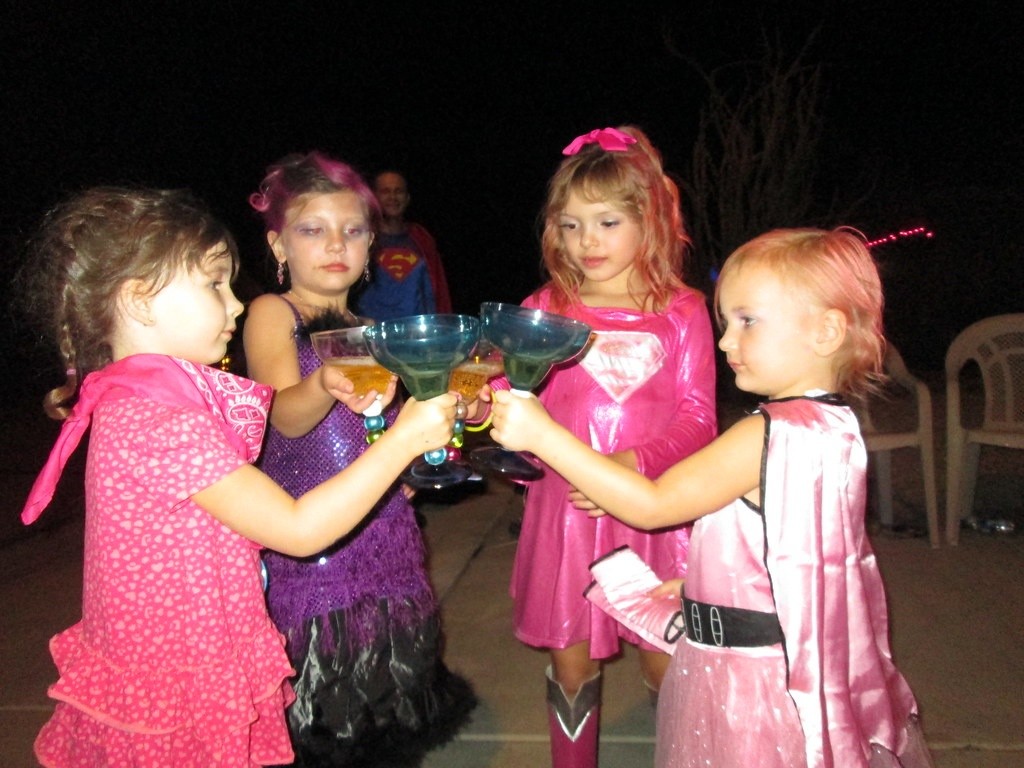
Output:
[363,315,480,491]
[449,329,503,481]
[465,301,593,480]
[309,326,397,445]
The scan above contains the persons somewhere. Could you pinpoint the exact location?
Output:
[14,185,458,768]
[344,171,451,329]
[243,154,474,768]
[489,227,934,768]
[446,125,717,768]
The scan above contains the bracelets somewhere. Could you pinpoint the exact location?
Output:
[464,386,498,432]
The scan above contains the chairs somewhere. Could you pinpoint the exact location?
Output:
[945,314,1024,547]
[849,340,940,549]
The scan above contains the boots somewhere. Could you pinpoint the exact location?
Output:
[643,681,659,709]
[545,664,600,768]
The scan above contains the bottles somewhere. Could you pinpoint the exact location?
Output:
[967,515,1015,536]
